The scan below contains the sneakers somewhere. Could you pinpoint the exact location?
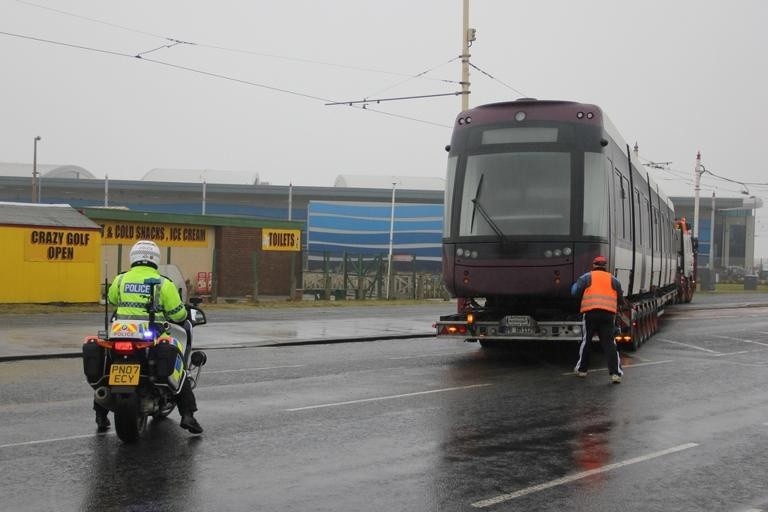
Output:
[179,415,204,434]
[575,369,588,377]
[96,417,112,429]
[611,373,621,384]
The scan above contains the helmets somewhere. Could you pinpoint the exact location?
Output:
[129,239,160,269]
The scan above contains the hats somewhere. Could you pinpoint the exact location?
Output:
[593,256,606,269]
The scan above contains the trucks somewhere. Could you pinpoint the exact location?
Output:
[432,218,699,353]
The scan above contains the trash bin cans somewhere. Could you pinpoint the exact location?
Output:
[743,275,757,289]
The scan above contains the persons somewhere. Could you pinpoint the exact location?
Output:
[92,241,203,433]
[570,255,625,383]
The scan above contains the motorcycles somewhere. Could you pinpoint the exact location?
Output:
[78,262,206,442]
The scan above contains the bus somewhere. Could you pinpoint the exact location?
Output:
[439,97,676,300]
